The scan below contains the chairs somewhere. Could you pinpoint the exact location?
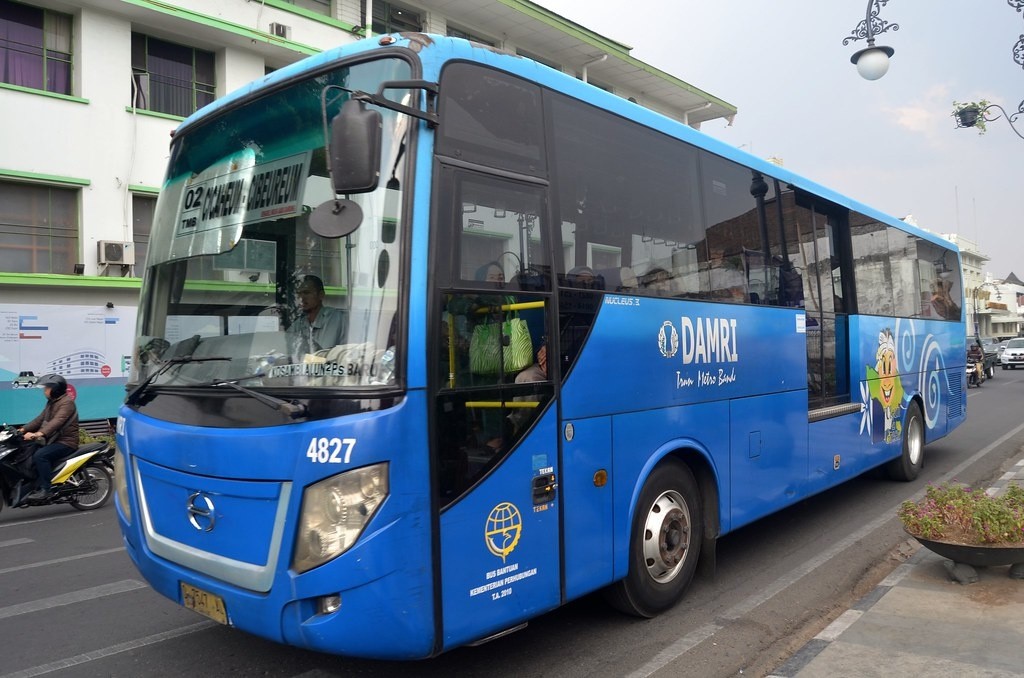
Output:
[507,265,633,368]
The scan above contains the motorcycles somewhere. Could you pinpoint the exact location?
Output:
[0,423,115,512]
[966,354,985,388]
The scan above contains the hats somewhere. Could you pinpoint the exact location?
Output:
[567,265,594,285]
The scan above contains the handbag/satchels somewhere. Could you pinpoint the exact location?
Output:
[468,294,531,374]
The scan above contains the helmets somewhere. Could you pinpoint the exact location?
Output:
[970,343,979,352]
[35,374,68,399]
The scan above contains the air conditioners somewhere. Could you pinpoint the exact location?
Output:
[269,23,292,40]
[97,240,135,265]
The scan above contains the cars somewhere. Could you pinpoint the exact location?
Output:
[1000,338,1024,370]
[980,337,1009,364]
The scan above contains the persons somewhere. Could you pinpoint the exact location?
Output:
[710,242,747,299]
[18,374,79,498]
[440,261,505,421]
[484,265,595,456]
[642,269,676,292]
[967,342,984,384]
[286,274,348,352]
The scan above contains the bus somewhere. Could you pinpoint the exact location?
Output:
[109,29,970,664]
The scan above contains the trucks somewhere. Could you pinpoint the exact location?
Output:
[967,347,996,379]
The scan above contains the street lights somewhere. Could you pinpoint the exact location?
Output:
[973,282,1002,345]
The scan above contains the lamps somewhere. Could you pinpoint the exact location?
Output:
[350,24,366,39]
[74,264,85,275]
[250,275,259,282]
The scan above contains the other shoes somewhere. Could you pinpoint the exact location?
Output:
[28,489,52,499]
